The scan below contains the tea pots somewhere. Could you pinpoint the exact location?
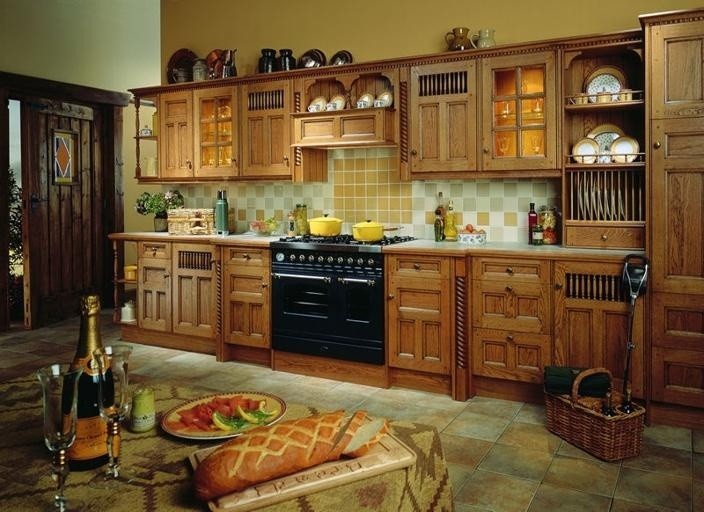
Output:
[444,25,496,52]
[173,66,188,83]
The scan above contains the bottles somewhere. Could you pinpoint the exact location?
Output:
[528,202,561,247]
[621,382,635,414]
[59,294,116,471]
[603,387,616,418]
[287,203,307,237]
[215,188,236,236]
[433,191,458,242]
[192,57,207,83]
[258,48,296,74]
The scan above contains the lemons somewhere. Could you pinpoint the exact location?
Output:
[212,413,232,431]
[259,401,277,415]
[238,405,258,423]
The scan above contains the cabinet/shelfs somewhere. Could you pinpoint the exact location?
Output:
[136,241,171,330]
[128,87,159,182]
[470,252,551,384]
[384,255,461,375]
[109,237,137,324]
[219,244,269,348]
[160,78,240,182]
[559,31,644,251]
[551,258,644,398]
[478,39,559,179]
[645,294,703,410]
[171,241,219,337]
[637,7,704,295]
[409,49,477,181]
[240,72,293,181]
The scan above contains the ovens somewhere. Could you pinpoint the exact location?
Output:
[271,241,387,367]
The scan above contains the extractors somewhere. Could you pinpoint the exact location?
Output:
[290,137,401,152]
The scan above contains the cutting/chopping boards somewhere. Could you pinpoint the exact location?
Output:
[187,413,419,512]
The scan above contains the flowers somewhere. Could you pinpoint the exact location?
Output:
[133,189,186,215]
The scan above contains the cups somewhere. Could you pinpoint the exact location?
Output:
[221,49,235,66]
[124,265,138,282]
[120,302,137,322]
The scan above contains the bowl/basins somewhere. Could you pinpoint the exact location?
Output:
[249,223,281,237]
[140,129,152,136]
[459,233,490,247]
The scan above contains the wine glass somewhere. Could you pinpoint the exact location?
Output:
[88,344,138,491]
[35,361,86,510]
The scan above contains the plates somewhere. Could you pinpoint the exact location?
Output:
[571,121,640,163]
[574,64,632,105]
[307,92,393,112]
[206,49,226,79]
[160,390,287,442]
[578,174,627,221]
[167,47,199,84]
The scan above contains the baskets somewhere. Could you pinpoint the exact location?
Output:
[166,207,236,236]
[542,366,647,463]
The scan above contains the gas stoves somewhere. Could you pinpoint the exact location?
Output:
[276,233,417,251]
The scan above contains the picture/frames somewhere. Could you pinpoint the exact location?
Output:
[51,126,81,185]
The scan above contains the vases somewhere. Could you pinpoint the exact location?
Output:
[153,211,168,232]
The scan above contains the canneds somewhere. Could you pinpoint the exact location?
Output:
[532,224,543,245]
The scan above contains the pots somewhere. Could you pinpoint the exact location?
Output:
[297,49,353,69]
[352,220,399,241]
[307,212,345,237]
